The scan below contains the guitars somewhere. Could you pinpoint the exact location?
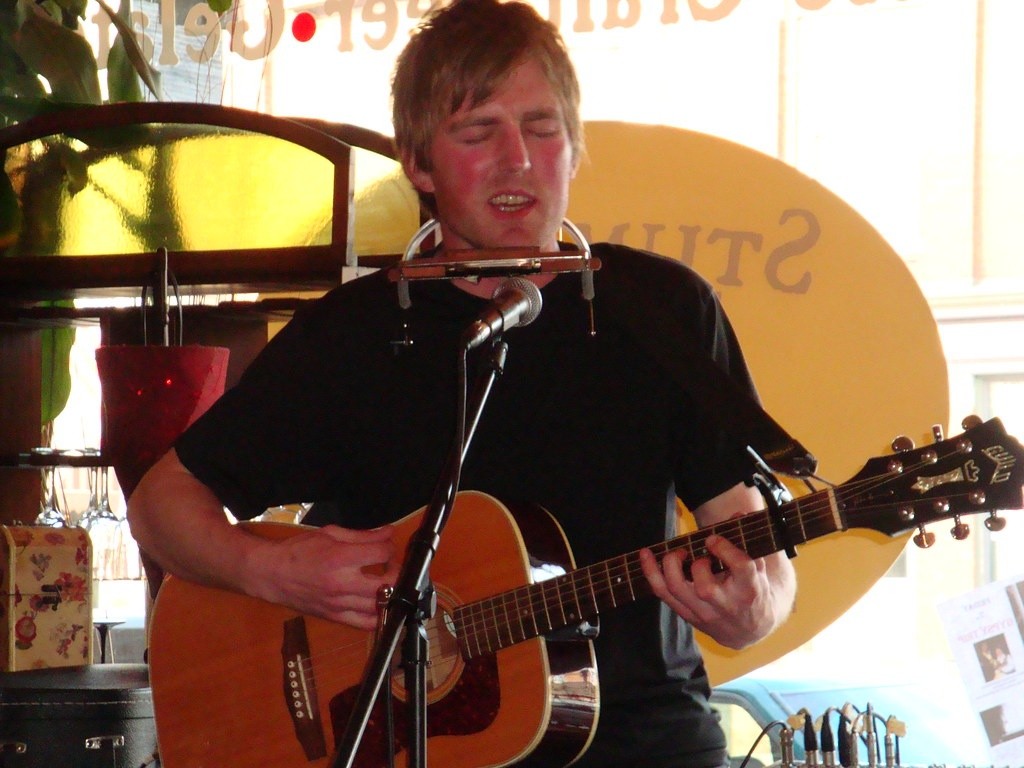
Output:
[145,415,1024,768]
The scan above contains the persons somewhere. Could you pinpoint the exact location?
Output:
[128,1,799,767]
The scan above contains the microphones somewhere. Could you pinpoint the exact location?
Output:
[458,278,543,353]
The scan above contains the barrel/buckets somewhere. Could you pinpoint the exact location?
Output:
[93,263,230,462]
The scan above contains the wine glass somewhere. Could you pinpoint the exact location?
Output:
[31,447,142,581]
